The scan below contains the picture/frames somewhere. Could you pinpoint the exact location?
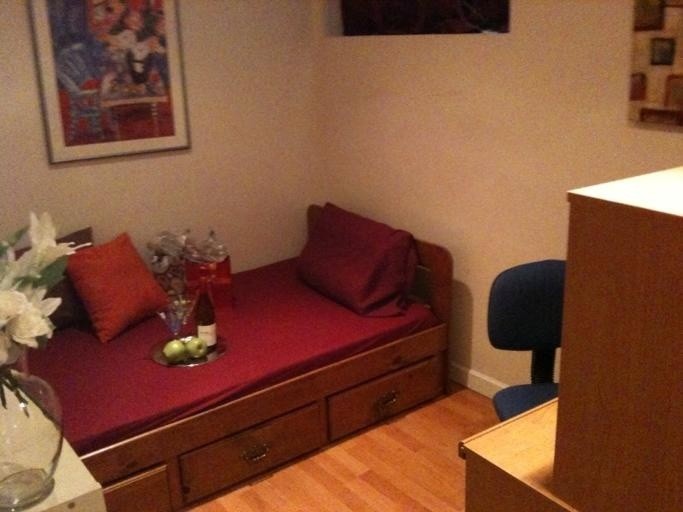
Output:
[27,0,191,166]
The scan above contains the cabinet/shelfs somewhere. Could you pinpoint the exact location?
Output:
[551,167,682,511]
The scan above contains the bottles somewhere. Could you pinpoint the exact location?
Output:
[195,277,217,352]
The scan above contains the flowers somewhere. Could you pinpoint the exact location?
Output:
[0,206,92,418]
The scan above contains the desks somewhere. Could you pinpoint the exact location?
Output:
[457,398,573,512]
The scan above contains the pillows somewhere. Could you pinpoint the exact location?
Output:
[295,201,416,318]
[15,226,170,346]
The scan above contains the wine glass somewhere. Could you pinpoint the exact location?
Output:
[156,293,195,339]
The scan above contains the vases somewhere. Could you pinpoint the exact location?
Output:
[0,367,64,512]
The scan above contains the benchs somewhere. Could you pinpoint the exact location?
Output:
[9,201,455,511]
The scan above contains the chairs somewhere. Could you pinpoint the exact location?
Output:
[486,257,566,421]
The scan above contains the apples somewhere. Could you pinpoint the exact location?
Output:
[162,339,186,364]
[185,338,208,359]
